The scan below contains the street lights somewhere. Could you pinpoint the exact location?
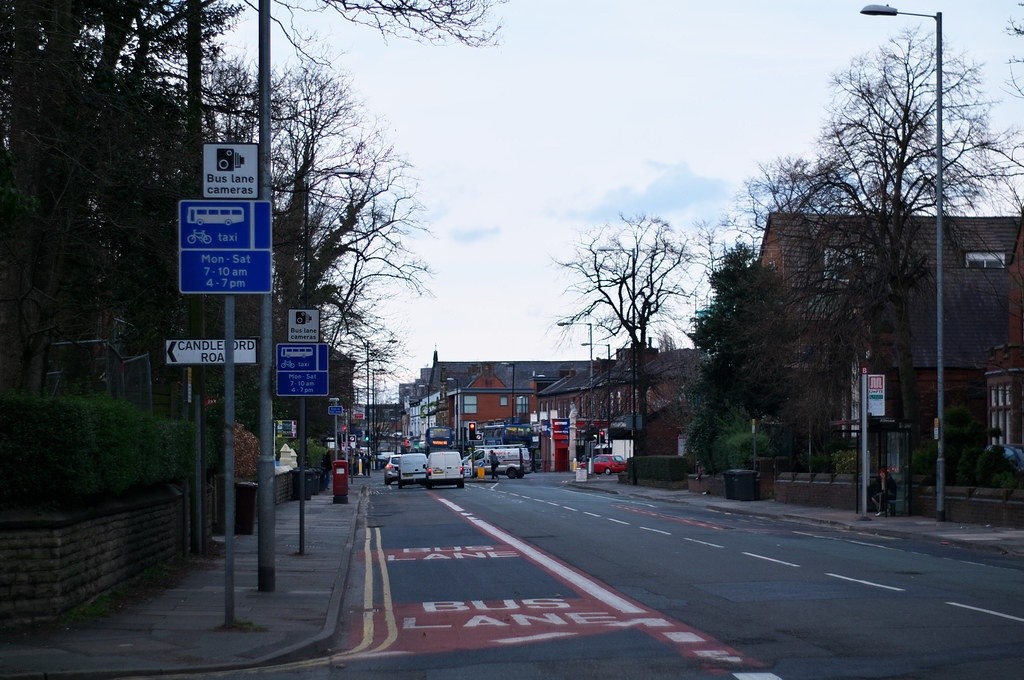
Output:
[418,384,430,427]
[296,169,359,458]
[556,322,611,453]
[860,4,950,523]
[447,378,459,448]
[501,362,514,425]
[365,340,399,475]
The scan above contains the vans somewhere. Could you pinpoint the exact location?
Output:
[462,448,532,480]
[398,453,428,489]
[427,452,465,489]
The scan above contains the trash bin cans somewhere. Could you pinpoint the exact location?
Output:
[234,483,258,535]
[290,467,322,502]
[721,469,736,499]
[736,470,761,501]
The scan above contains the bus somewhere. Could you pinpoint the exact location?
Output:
[474,425,532,448]
[425,426,453,457]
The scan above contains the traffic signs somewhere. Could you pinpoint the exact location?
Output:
[164,340,257,363]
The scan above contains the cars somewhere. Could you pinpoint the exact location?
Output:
[986,445,1024,474]
[580,453,627,476]
[384,455,401,484]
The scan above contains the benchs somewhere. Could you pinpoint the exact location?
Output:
[887,499,904,517]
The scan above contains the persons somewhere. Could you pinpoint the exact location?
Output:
[324,450,333,488]
[490,450,499,481]
[357,453,367,476]
[871,468,897,517]
[569,402,578,424]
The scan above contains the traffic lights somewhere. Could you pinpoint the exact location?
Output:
[600,430,606,444]
[588,423,596,442]
[365,430,369,441]
[468,422,483,441]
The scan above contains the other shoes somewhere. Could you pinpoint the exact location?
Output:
[497,477,499,481]
[874,511,886,516]
